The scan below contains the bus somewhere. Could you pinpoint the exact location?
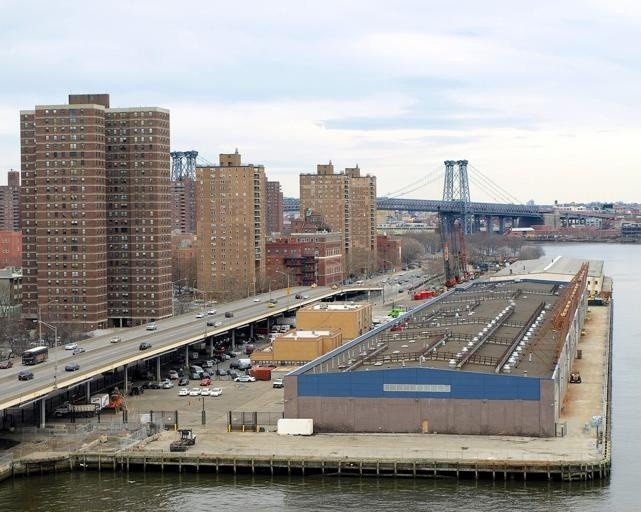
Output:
[21,345,49,366]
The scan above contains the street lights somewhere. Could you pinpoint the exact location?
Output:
[198,396,206,425]
[382,280,386,303]
[269,279,276,303]
[276,270,291,305]
[33,319,59,388]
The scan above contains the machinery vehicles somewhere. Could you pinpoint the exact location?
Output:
[177,428,197,445]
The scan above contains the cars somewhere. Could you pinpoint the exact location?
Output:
[169,440,189,452]
[254,294,310,308]
[111,335,122,343]
[311,283,318,287]
[447,262,492,288]
[65,362,80,371]
[127,308,297,396]
[74,347,86,353]
[65,342,78,350]
[31,334,63,347]
[0,360,13,369]
[332,266,440,293]
[19,370,33,381]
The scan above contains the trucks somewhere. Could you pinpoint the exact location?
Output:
[91,393,110,414]
[54,401,97,418]
